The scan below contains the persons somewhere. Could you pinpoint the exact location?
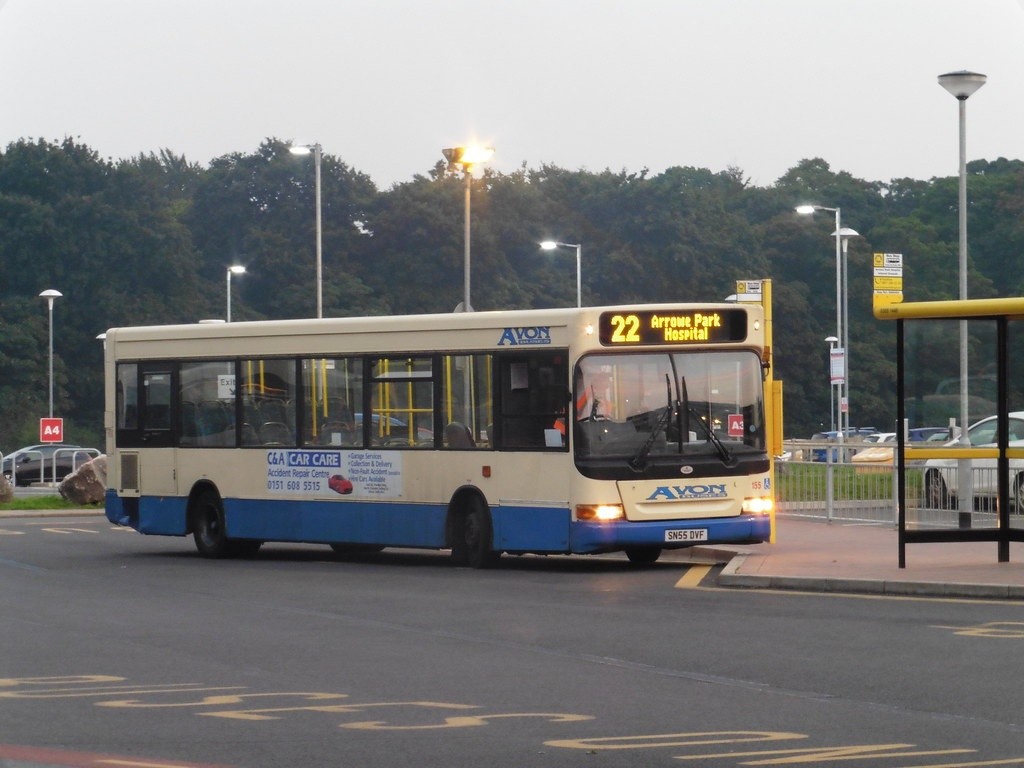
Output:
[553,372,614,436]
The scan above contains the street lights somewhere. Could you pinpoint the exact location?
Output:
[442,146,492,314]
[829,226,860,461]
[795,203,845,463]
[935,68,987,529]
[225,264,247,375]
[40,289,64,445]
[538,239,582,308]
[290,143,322,401]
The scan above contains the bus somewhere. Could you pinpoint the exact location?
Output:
[103,301,771,571]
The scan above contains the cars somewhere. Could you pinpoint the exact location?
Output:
[922,411,1024,510]
[353,412,432,442]
[773,424,961,477]
[3,443,94,487]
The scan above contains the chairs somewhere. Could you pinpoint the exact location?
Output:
[180,396,477,451]
